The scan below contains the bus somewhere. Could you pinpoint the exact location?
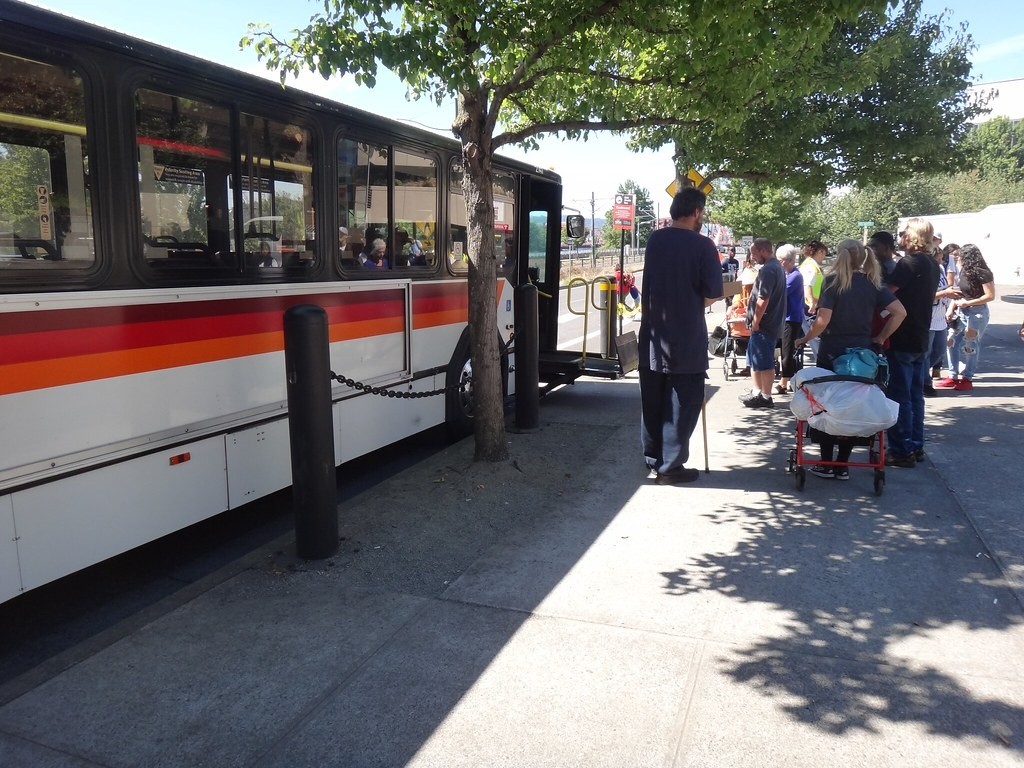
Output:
[0,0,585,611]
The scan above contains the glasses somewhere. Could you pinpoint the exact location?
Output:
[898,231,906,237]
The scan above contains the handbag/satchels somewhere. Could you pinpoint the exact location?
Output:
[832,346,878,381]
[708,326,731,358]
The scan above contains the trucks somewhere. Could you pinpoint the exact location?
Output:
[794,247,805,266]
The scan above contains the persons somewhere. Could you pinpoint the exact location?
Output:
[243,224,428,271]
[722,217,996,478]
[636,186,725,484]
[613,265,631,318]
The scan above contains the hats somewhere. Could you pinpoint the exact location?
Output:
[934,232,943,244]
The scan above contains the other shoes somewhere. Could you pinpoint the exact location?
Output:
[808,459,851,480]
[738,388,775,408]
[871,446,927,468]
[923,386,937,396]
[934,376,958,387]
[771,384,788,394]
[647,463,699,484]
[953,376,974,390]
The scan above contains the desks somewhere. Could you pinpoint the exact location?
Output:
[704,281,743,314]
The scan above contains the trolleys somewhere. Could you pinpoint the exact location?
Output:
[722,288,786,383]
[791,344,892,495]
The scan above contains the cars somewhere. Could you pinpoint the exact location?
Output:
[715,246,726,253]
